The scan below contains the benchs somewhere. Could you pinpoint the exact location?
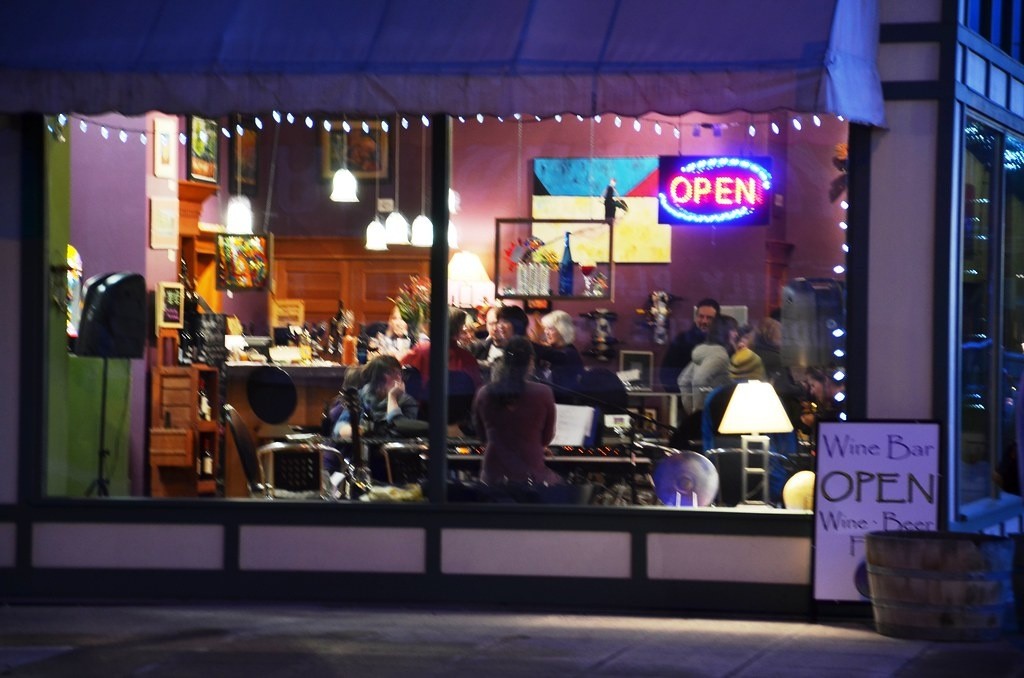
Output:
[420,479,598,506]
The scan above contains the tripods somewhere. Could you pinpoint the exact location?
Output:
[83,358,113,497]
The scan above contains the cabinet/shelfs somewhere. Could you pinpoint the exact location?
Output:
[145,329,220,499]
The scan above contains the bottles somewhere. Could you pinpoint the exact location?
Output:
[560,231,573,296]
[299,300,371,366]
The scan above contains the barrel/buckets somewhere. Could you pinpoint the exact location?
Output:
[864,530,1019,644]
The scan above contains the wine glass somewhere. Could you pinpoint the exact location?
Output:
[579,256,598,297]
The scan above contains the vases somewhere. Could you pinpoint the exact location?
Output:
[867,528,1020,642]
[410,334,420,352]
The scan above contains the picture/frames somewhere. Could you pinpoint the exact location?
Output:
[619,349,654,393]
[156,282,185,329]
[214,233,271,291]
[187,112,221,187]
[315,114,393,186]
[228,111,260,201]
[153,113,180,180]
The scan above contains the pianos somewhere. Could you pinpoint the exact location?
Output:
[415,435,654,507]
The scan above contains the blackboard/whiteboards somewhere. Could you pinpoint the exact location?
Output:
[812,415,944,607]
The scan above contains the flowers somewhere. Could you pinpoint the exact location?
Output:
[384,272,434,336]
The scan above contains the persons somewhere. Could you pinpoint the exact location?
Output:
[802,362,847,475]
[317,303,629,505]
[659,298,813,507]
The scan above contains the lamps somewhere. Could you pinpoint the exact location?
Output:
[217,114,462,250]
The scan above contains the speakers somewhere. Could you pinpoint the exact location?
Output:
[73,271,146,358]
[781,276,845,367]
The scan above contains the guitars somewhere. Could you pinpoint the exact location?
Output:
[340,385,373,502]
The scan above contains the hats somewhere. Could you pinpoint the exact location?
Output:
[727,347,764,381]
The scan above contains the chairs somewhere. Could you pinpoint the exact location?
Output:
[219,402,268,498]
[399,363,801,512]
